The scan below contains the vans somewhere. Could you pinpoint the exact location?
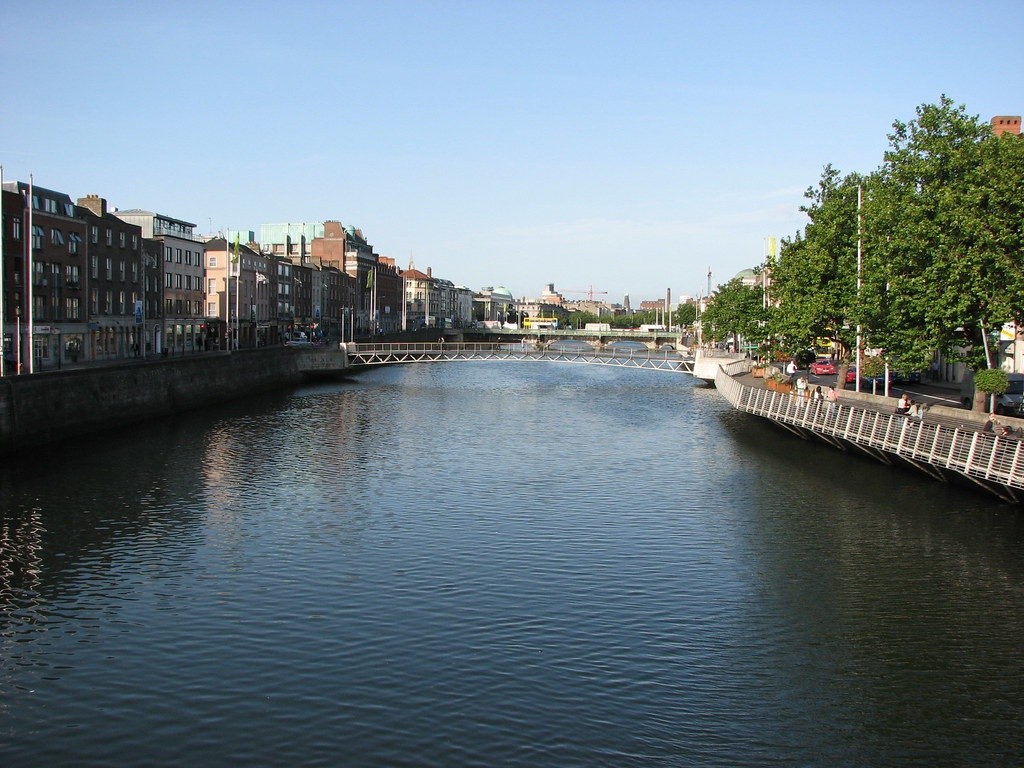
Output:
[960,367,1024,419]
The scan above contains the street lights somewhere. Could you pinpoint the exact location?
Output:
[846,184,861,392]
[15,306,22,375]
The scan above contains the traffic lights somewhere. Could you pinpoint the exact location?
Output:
[311,325,313,330]
[201,324,204,332]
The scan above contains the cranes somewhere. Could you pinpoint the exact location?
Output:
[559,284,607,302]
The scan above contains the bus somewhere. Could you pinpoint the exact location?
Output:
[521,317,558,329]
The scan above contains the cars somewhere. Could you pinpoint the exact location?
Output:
[809,359,835,375]
[839,366,856,383]
[890,370,921,384]
[860,373,892,390]
[292,332,308,341]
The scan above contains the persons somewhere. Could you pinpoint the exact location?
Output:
[982,414,1023,439]
[895,393,927,424]
[797,376,805,396]
[437,336,445,343]
[497,336,504,342]
[786,360,798,378]
[827,385,837,401]
[814,385,824,400]
